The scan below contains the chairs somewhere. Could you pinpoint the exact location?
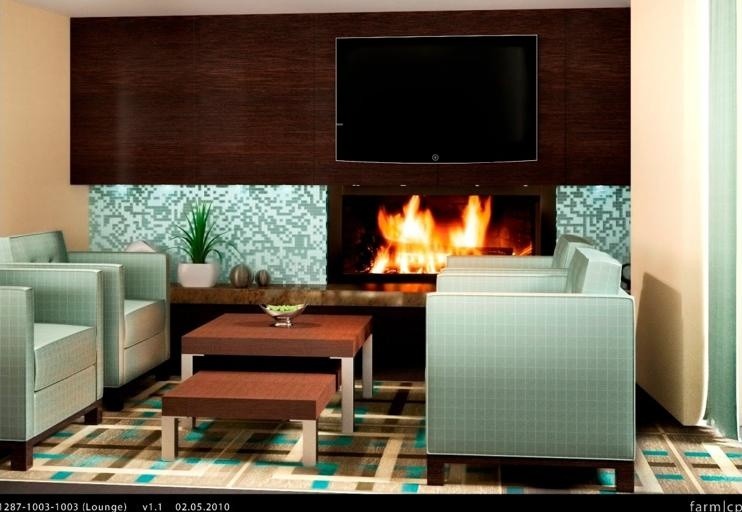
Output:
[0,229,173,472]
[424,233,636,493]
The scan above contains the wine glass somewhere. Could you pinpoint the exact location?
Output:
[258,303,309,327]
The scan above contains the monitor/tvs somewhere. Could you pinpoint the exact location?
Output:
[334,33,541,167]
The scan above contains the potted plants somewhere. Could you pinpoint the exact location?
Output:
[169,201,247,289]
